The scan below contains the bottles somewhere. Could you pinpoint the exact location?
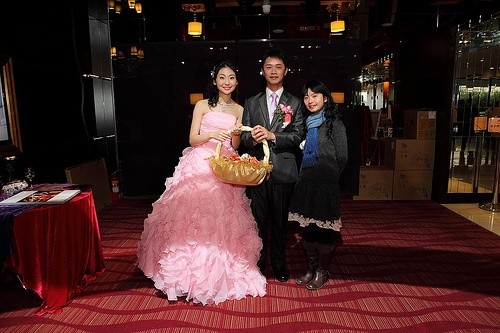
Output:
[359,52,394,88]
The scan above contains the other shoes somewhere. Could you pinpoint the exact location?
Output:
[295,271,315,286]
[306,268,330,289]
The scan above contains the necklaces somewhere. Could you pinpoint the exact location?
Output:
[217,100,231,112]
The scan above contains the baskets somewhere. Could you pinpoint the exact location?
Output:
[209,126,270,186]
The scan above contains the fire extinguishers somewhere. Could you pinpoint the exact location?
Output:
[111,172,121,203]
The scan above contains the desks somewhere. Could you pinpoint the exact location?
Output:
[0,180,107,311]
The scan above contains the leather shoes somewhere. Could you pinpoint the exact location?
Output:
[272,263,288,281]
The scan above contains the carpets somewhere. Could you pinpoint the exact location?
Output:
[0,195,500,333]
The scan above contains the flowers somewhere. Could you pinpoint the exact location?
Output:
[219,150,260,166]
[274,100,294,130]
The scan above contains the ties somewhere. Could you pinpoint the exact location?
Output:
[270,93,278,121]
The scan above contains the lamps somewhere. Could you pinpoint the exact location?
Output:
[325,0,345,33]
[182,2,205,36]
[262,1,271,14]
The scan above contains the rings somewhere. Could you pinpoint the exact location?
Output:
[235,130,238,133]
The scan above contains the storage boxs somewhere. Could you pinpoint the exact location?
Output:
[352,165,393,201]
[393,169,434,200]
[394,138,436,170]
[404,107,436,141]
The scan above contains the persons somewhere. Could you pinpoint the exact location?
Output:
[287,81,348,289]
[240,49,305,282]
[134,61,268,306]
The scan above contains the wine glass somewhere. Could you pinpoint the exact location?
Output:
[25,167,36,189]
